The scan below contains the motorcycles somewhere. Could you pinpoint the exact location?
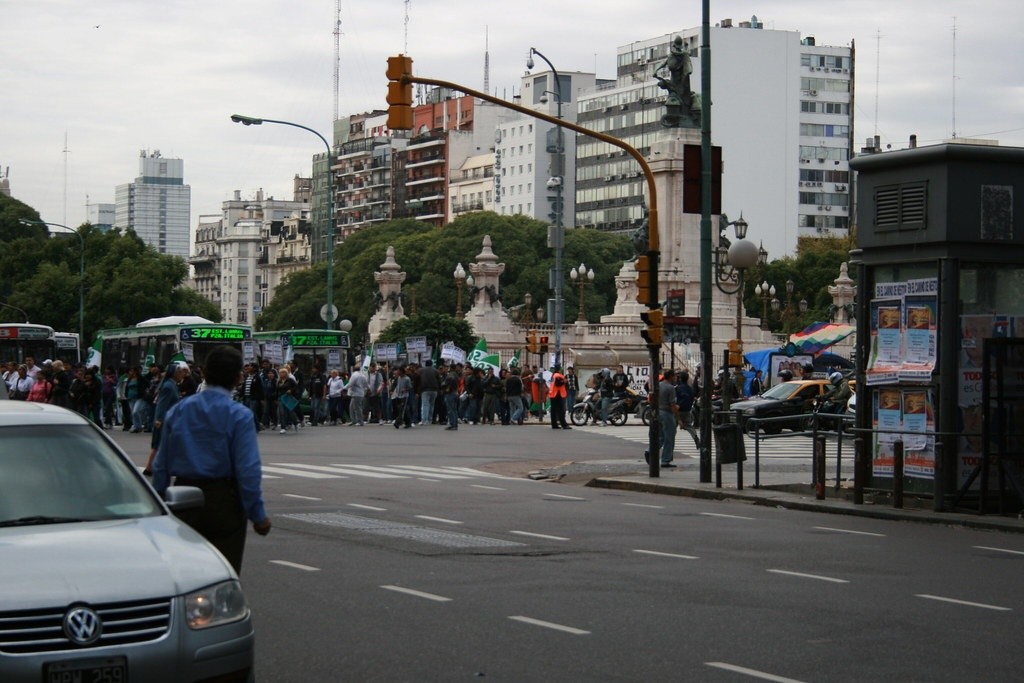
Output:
[800,394,854,433]
[570,386,630,426]
[640,391,748,428]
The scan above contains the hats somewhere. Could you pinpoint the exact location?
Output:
[43,359,52,367]
[51,360,65,372]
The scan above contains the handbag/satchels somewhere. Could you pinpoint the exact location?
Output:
[14,390,29,400]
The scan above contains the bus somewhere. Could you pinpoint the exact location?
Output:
[253,329,350,414]
[97,316,253,375]
[0,323,81,366]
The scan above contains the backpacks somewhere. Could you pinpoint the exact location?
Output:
[726,380,740,398]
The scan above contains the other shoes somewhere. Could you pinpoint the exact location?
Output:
[98,413,576,433]
[599,421,607,426]
[661,462,677,467]
[143,470,153,476]
[644,450,650,464]
[589,421,596,426]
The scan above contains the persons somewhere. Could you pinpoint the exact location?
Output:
[175,360,206,397]
[373,290,399,312]
[547,362,572,430]
[142,364,184,476]
[613,365,629,396]
[748,370,766,395]
[643,364,701,468]
[150,345,273,577]
[564,367,580,414]
[823,372,851,431]
[231,359,546,434]
[467,284,496,308]
[655,36,693,103]
[0,354,159,434]
[778,361,803,376]
[590,368,614,427]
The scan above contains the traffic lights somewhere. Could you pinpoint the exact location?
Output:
[525,329,537,353]
[540,337,549,354]
[640,309,664,346]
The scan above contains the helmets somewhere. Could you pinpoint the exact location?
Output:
[718,369,730,380]
[801,361,813,377]
[602,368,610,378]
[695,362,701,374]
[829,371,843,385]
[778,369,793,379]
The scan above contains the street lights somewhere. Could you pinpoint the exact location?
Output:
[525,292,545,330]
[569,262,595,320]
[755,281,776,331]
[453,263,474,321]
[231,114,333,330]
[717,210,768,392]
[19,218,84,346]
[771,278,807,344]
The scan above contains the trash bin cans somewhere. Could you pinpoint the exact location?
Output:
[711,411,748,464]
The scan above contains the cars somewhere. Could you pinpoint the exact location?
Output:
[730,378,856,435]
[0,400,255,683]
